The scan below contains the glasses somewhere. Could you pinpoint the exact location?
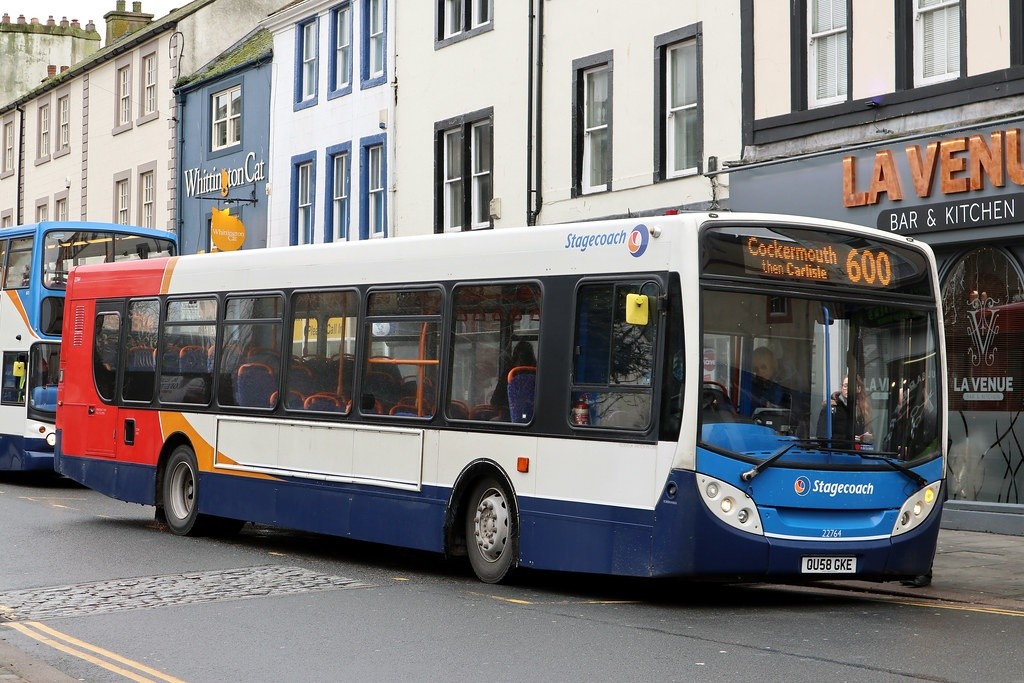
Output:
[842,383,849,390]
[753,364,772,371]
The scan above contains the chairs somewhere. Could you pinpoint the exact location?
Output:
[703,380,738,414]
[101,341,537,424]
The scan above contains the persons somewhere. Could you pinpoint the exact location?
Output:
[490,341,537,422]
[816,374,871,449]
[881,391,953,587]
[750,347,807,414]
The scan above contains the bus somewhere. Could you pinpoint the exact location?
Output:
[54,207,951,587]
[1,221,178,475]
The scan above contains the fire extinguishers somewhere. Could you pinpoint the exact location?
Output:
[573,393,590,424]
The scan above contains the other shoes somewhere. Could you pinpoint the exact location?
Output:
[913,572,931,587]
